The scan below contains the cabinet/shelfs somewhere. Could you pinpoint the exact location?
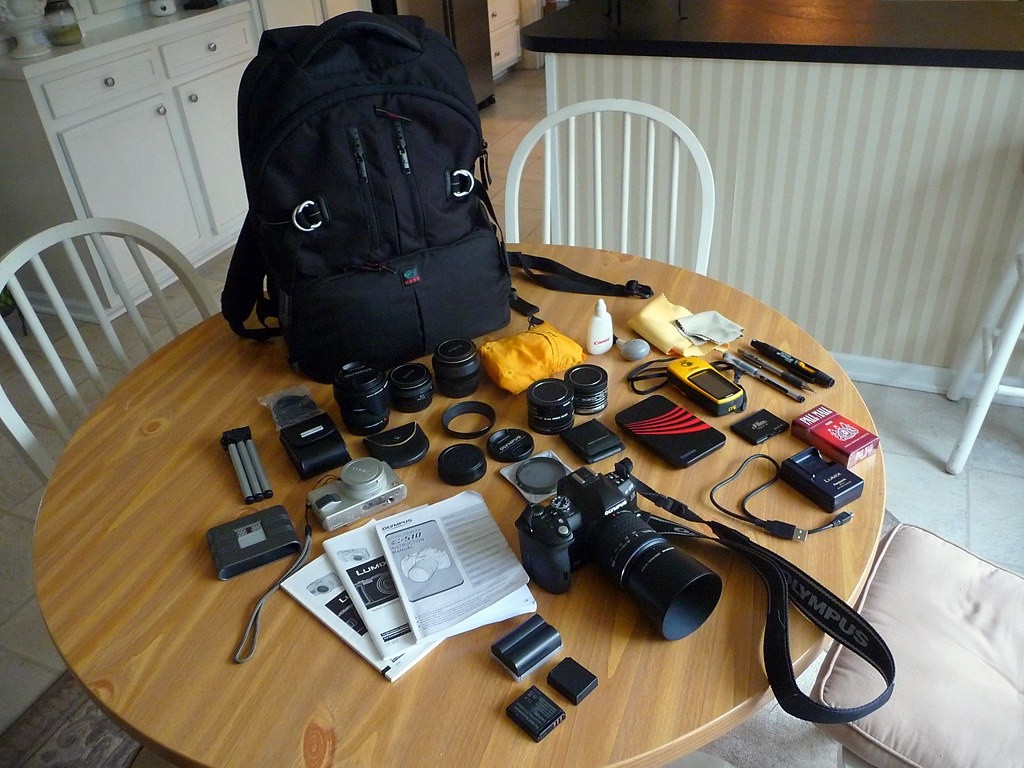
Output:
[452,0,522,82]
[0,0,265,324]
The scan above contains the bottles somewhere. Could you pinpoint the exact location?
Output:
[586,299,613,356]
[43,0,82,45]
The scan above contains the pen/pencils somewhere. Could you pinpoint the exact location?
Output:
[737,348,815,393]
[750,339,836,388]
[722,352,805,403]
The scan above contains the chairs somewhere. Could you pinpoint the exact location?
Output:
[810,523,1024,768]
[506,98,715,277]
[0,218,216,486]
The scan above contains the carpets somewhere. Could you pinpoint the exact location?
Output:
[0,509,897,768]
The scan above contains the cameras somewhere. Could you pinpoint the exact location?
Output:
[513,466,723,642]
[309,457,407,532]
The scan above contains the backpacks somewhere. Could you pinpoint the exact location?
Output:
[219,9,513,383]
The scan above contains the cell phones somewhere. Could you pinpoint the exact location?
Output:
[667,355,745,418]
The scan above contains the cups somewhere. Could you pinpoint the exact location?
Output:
[147,1,177,16]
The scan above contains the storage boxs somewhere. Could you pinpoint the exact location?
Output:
[791,404,880,469]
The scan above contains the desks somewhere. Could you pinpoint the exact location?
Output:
[34,243,887,766]
